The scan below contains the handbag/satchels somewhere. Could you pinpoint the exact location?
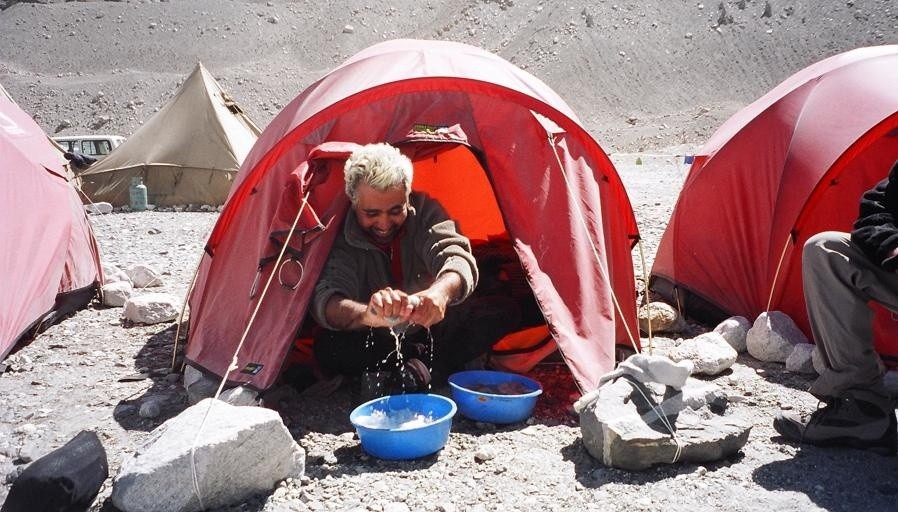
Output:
[462,313,558,370]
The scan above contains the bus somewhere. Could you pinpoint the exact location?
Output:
[50,135,127,163]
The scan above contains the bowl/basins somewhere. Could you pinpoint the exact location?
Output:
[350,393,457,460]
[448,370,544,425]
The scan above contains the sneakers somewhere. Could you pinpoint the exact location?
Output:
[361,372,390,399]
[774,384,896,446]
[402,358,436,390]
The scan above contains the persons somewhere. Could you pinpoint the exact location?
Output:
[772,160,898,446]
[309,142,481,390]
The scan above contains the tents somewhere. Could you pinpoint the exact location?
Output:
[183,36,643,399]
[79,60,263,206]
[0,82,104,362]
[650,42,898,358]
